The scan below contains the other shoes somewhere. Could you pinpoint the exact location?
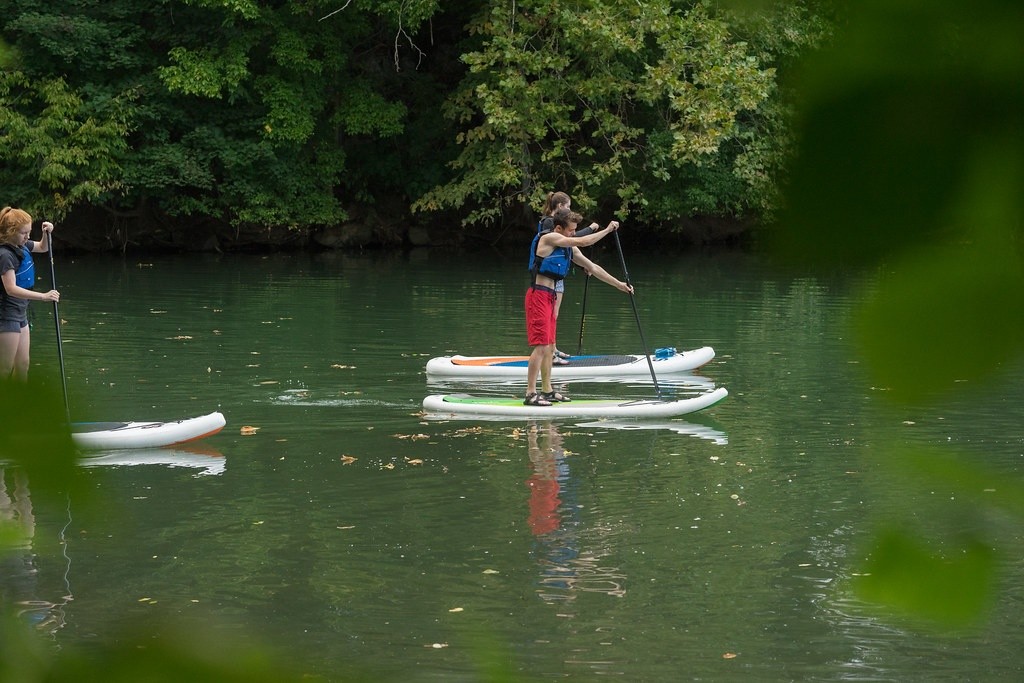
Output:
[553,354,569,365]
[555,350,570,358]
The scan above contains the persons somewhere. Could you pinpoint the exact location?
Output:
[0,207,60,381]
[524,210,634,405]
[538,192,599,364]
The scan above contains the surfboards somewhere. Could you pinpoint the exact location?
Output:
[62,412,227,452]
[423,387,729,418]
[425,346,715,377]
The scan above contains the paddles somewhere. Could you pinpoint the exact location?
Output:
[614,228,663,400]
[47,231,72,440]
[578,231,595,355]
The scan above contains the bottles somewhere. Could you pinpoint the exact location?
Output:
[655,347,677,360]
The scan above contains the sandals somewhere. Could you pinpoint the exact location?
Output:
[523,393,552,406]
[540,391,571,402]
